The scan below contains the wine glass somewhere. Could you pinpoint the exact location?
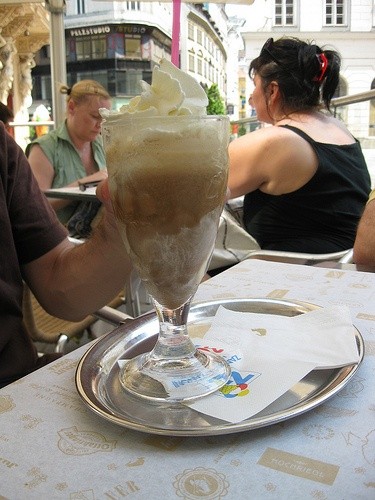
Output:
[99,112,231,406]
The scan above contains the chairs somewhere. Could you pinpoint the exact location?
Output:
[22,281,129,355]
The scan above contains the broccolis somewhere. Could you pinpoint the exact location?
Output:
[200,81,224,115]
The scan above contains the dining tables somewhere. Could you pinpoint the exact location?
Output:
[0,259,375,500]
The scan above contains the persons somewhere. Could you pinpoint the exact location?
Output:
[25,79,111,242]
[0,37,375,390]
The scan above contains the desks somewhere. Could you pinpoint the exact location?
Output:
[44,186,245,214]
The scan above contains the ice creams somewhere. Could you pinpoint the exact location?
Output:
[96,57,230,311]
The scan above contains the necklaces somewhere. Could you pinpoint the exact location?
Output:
[281,107,308,119]
